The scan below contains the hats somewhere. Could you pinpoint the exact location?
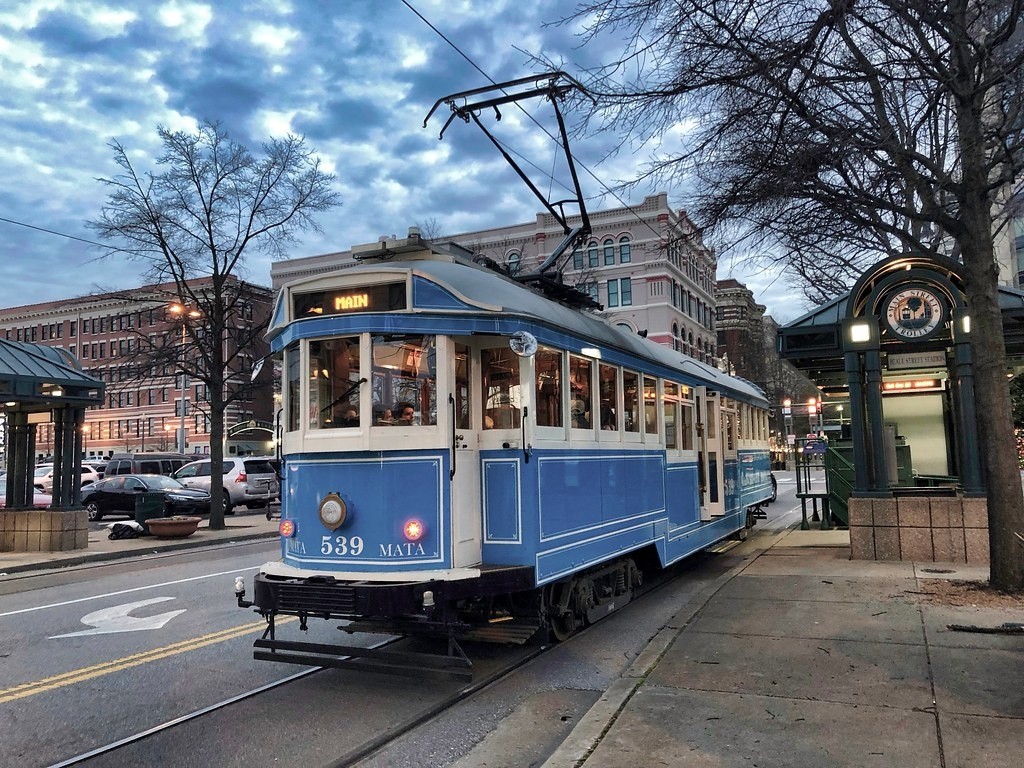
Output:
[398,402,414,418]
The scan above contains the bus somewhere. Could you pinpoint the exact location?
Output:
[235,225,778,685]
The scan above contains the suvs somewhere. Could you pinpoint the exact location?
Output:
[0,452,282,515]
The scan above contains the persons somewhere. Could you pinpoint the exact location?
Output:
[571,398,618,431]
[337,405,357,421]
[372,402,414,426]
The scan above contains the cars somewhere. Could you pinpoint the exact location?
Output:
[80,474,211,521]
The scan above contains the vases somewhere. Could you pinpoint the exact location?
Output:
[145,517,202,539]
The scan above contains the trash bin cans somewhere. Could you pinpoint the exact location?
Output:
[133,491,166,536]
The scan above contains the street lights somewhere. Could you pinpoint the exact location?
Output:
[169,305,202,454]
[164,424,172,452]
[82,424,90,452]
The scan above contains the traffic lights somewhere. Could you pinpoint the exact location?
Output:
[816,402,822,414]
